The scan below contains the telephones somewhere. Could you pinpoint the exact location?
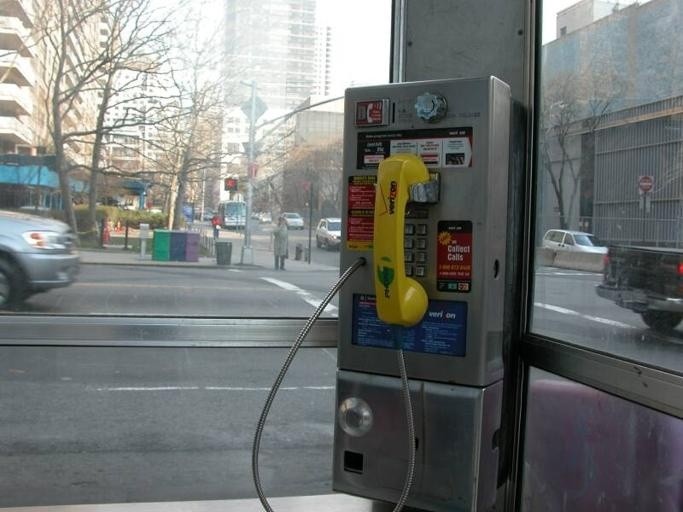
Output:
[337,76,525,388]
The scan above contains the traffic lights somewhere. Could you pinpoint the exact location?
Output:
[224,177,238,191]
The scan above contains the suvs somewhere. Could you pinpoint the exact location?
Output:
[280,211,304,231]
[316,216,342,251]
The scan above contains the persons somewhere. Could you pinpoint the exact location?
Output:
[273,217,289,272]
[211,214,221,239]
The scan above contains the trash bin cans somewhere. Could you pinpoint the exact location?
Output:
[215,242,232,265]
[153,229,200,262]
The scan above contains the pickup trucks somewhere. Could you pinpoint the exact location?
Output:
[594,242,683,330]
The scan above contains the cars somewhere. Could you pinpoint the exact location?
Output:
[251,211,273,223]
[0,209,81,310]
[195,206,218,222]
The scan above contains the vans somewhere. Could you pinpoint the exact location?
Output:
[542,228,609,256]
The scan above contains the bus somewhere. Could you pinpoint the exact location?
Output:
[218,201,247,230]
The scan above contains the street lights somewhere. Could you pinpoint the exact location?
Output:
[304,199,312,264]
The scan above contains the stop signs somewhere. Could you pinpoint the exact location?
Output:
[637,174,655,197]
